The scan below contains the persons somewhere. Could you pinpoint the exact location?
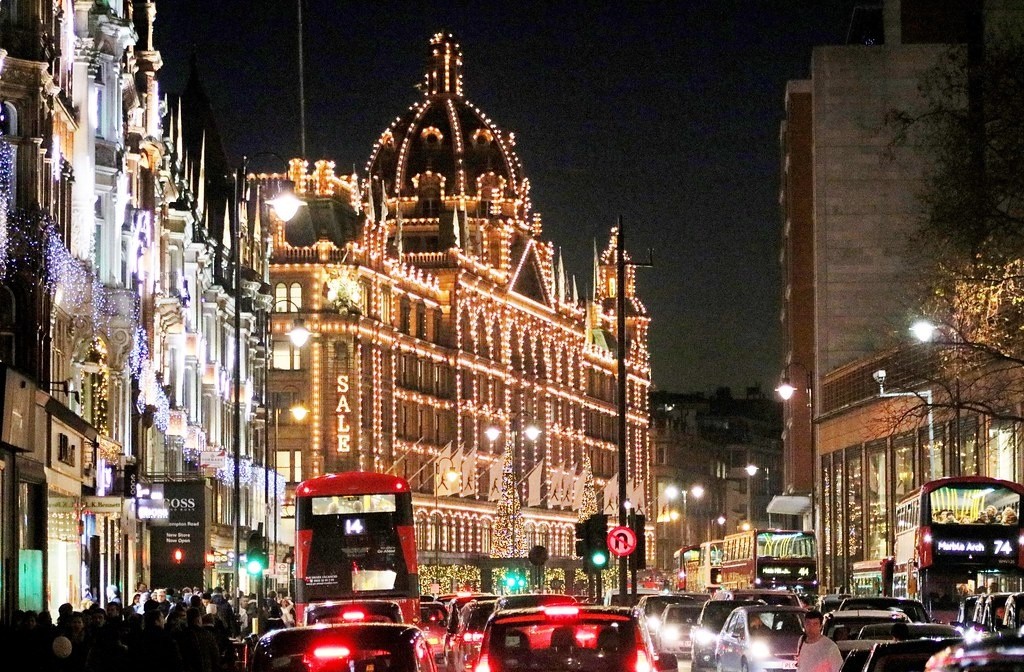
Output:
[353,501,362,512]
[747,614,759,631]
[934,505,1017,525]
[795,609,844,672]
[0,582,293,672]
[958,583,1001,600]
[328,502,339,514]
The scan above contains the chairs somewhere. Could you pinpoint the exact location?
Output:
[597,627,619,652]
[506,629,532,651]
[551,627,575,653]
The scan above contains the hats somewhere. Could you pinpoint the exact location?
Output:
[206,604,216,615]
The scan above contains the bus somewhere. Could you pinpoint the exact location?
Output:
[671,544,702,595]
[282,471,423,629]
[696,538,724,595]
[890,476,1024,624]
[718,526,820,603]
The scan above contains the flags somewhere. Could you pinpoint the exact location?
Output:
[562,465,576,506]
[657,491,669,522]
[458,447,475,498]
[550,461,565,505]
[603,474,618,513]
[487,455,504,502]
[528,462,541,506]
[630,481,645,515]
[572,471,586,510]
[446,445,464,497]
[618,479,634,515]
[434,442,452,497]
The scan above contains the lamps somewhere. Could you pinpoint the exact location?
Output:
[50,389,80,404]
[50,381,68,397]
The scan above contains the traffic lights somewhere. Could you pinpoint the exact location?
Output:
[246,530,264,576]
[175,548,182,565]
[575,521,590,573]
[589,513,609,570]
[205,553,215,565]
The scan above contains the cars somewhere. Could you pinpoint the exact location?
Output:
[244,583,1024,672]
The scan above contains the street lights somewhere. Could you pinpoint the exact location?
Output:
[273,384,312,591]
[666,484,704,545]
[910,318,961,478]
[744,460,772,529]
[264,298,313,605]
[485,409,543,558]
[232,151,305,616]
[776,362,816,531]
